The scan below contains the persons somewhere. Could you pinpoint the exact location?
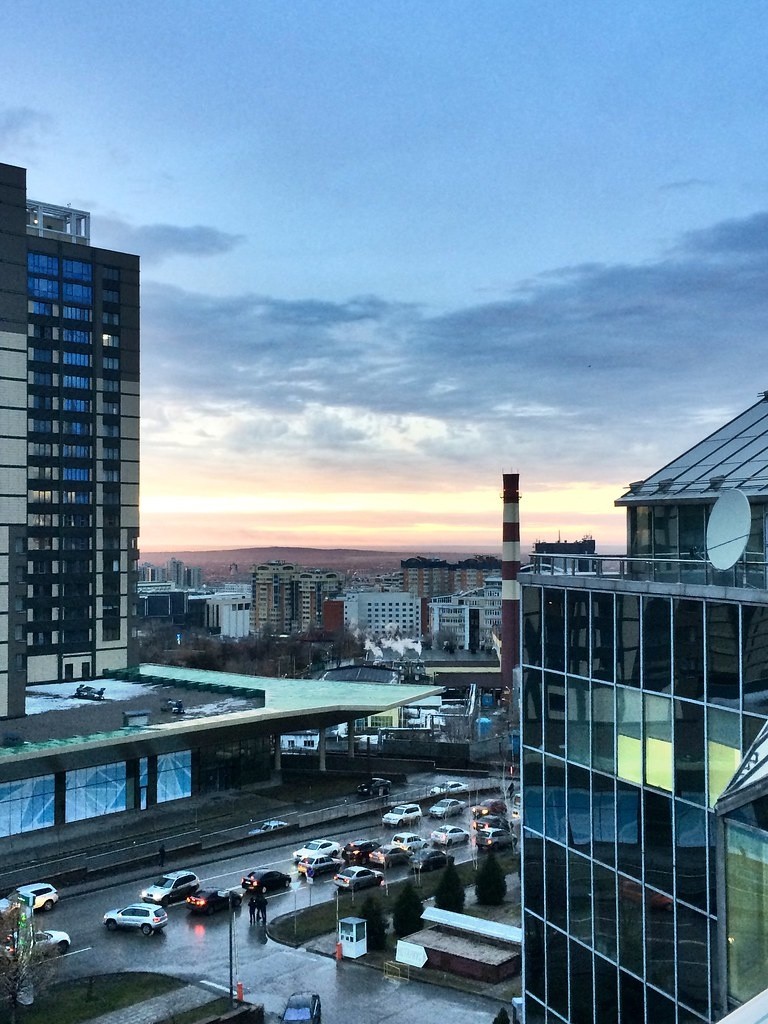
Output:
[248,895,268,924]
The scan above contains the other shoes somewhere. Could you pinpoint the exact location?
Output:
[158,861,166,866]
[250,917,267,924]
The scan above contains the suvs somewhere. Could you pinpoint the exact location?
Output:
[0,883,60,918]
[102,903,169,936]
[140,870,200,907]
[381,804,423,828]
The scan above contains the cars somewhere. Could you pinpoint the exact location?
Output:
[429,781,470,797]
[241,868,292,895]
[475,827,519,852]
[369,844,414,868]
[5,930,72,960]
[389,832,429,852]
[427,798,466,820]
[406,849,455,874]
[333,866,384,891]
[355,778,392,796]
[470,800,507,818]
[430,825,469,847]
[278,992,322,1024]
[246,820,287,835]
[341,839,382,867]
[184,887,242,915]
[472,815,515,833]
[292,839,342,864]
[297,854,343,878]
